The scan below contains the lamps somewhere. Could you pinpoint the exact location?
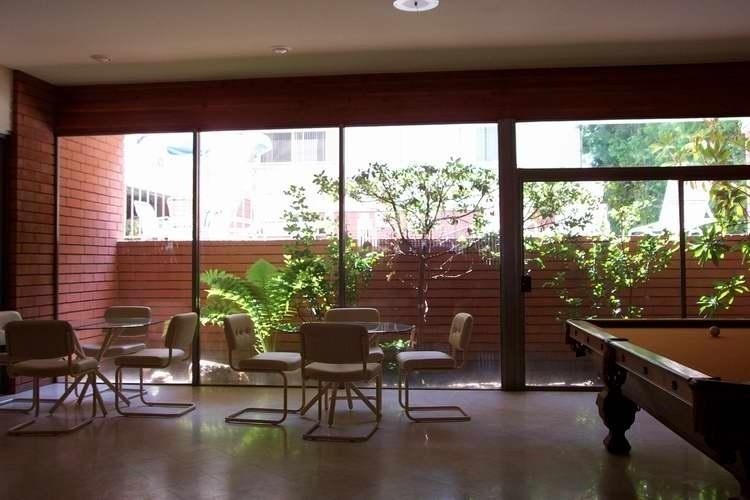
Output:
[392,0,440,13]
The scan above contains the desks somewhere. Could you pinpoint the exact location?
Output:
[563,317,750,499]
[48,317,165,417]
[300,322,413,425]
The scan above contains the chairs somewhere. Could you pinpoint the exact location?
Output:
[299,322,383,442]
[75,305,152,400]
[397,313,474,422]
[324,308,380,411]
[224,314,305,424]
[115,312,199,416]
[0,311,68,411]
[4,319,99,435]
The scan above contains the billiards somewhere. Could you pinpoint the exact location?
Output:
[709,325,722,337]
